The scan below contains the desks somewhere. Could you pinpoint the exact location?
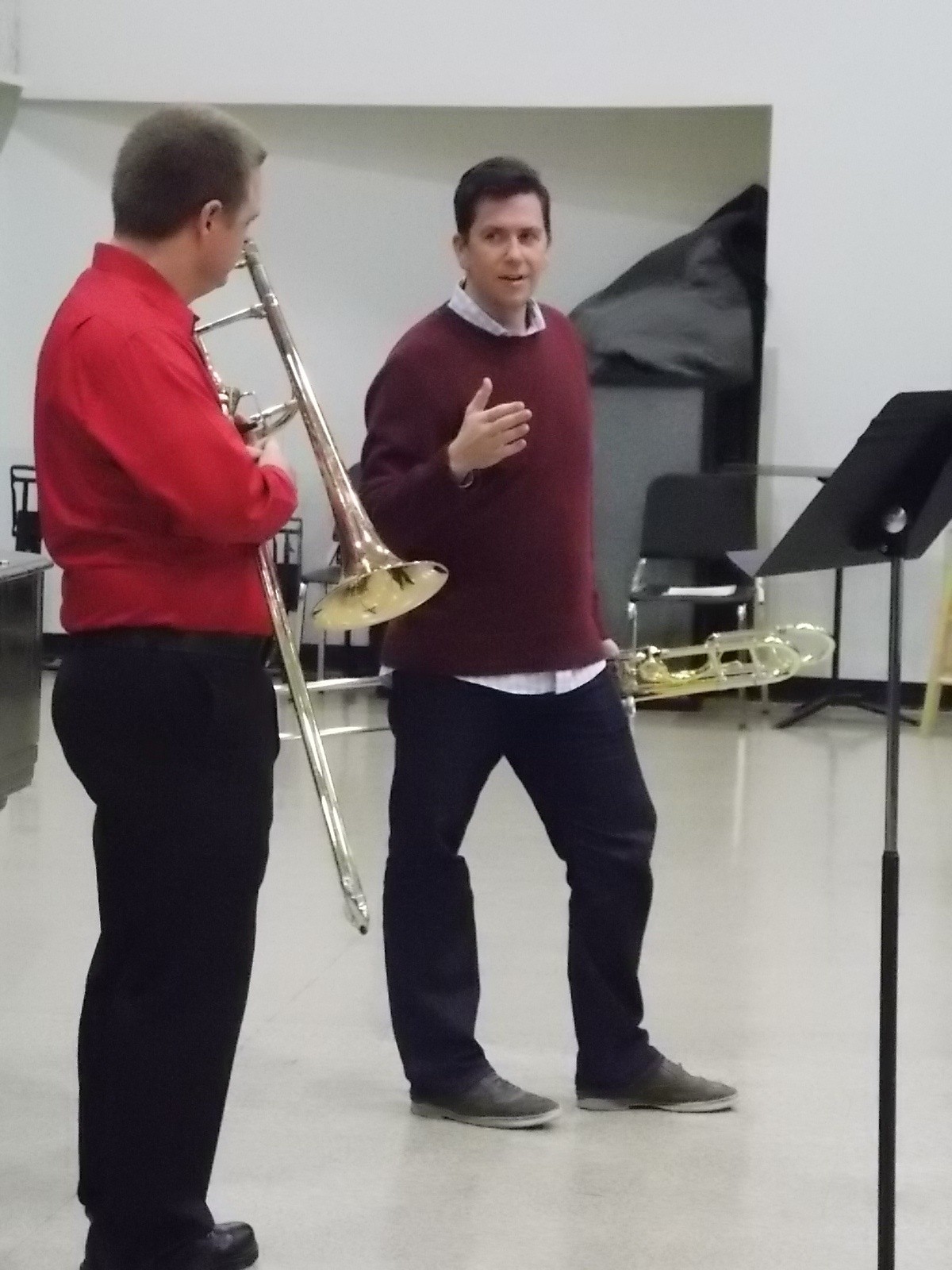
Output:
[0,550,54,810]
[301,569,368,693]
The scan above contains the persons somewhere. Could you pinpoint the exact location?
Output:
[33,107,301,1270]
[358,158,736,1128]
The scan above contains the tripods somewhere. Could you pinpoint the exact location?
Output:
[775,569,920,732]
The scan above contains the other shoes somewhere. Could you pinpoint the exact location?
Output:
[411,1072,560,1128]
[573,1048,737,1111]
[79,1220,258,1270]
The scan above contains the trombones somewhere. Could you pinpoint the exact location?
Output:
[183,234,456,940]
[276,621,845,746]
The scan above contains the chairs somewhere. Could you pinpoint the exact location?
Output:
[626,474,769,727]
